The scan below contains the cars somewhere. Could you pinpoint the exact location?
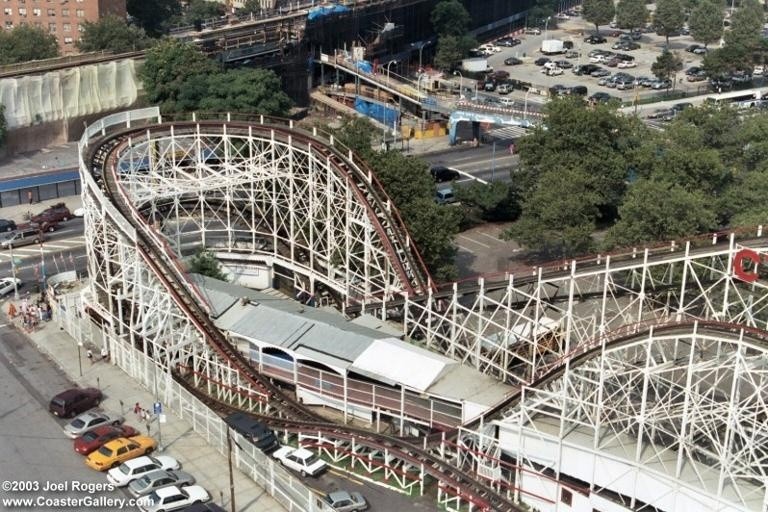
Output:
[0,207,84,248]
[535,32,671,104]
[436,188,456,203]
[492,71,509,78]
[504,58,522,65]
[63,412,227,512]
[431,167,460,182]
[648,103,693,120]
[469,37,521,56]
[525,9,579,35]
[686,46,707,81]
[323,492,367,512]
[272,447,327,477]
[225,412,278,453]
[753,67,767,75]
[0,277,22,297]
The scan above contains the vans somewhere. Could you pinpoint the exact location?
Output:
[497,83,513,94]
[499,97,515,106]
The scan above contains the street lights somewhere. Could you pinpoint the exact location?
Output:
[387,59,397,82]
[419,41,431,66]
[453,70,463,97]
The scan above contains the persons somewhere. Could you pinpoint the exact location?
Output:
[508,143,515,156]
[135,402,149,423]
[9,297,52,334]
[28,189,33,204]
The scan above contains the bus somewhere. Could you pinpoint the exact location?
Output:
[481,316,565,359]
[705,92,757,112]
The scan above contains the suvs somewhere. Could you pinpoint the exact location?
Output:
[49,388,102,417]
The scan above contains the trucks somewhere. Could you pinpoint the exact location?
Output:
[485,80,496,91]
[462,57,493,73]
